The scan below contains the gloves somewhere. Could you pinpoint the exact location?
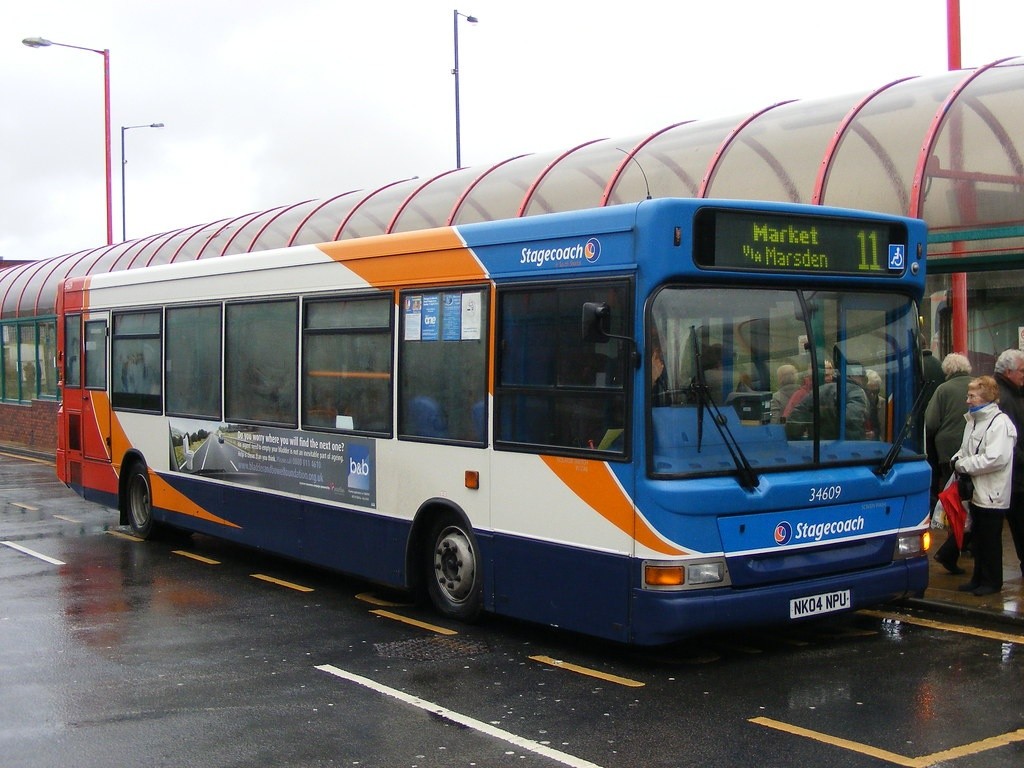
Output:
[950,456,959,470]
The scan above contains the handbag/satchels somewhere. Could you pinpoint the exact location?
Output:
[957,473,975,501]
[930,467,971,533]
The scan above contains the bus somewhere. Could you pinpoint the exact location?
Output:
[55,196,934,647]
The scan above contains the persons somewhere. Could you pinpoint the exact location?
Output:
[920,332,1024,596]
[701,343,741,406]
[771,360,883,441]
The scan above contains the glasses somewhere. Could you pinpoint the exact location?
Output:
[965,393,980,401]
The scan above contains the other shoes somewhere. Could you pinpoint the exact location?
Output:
[973,585,1002,595]
[934,552,966,574]
[957,582,976,591]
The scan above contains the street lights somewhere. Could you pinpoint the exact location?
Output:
[122,123,164,241]
[22,36,112,245]
[452,10,479,168]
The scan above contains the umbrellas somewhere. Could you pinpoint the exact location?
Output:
[938,460,967,558]
